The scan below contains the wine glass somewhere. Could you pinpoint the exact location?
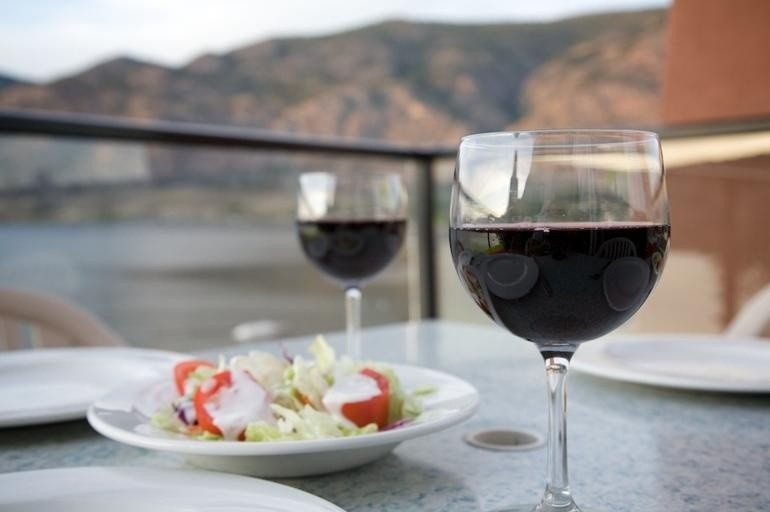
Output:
[296,171,408,356]
[448,129,673,512]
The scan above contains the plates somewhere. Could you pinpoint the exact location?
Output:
[1,466,346,511]
[0,345,192,428]
[571,332,770,393]
[87,362,481,477]
